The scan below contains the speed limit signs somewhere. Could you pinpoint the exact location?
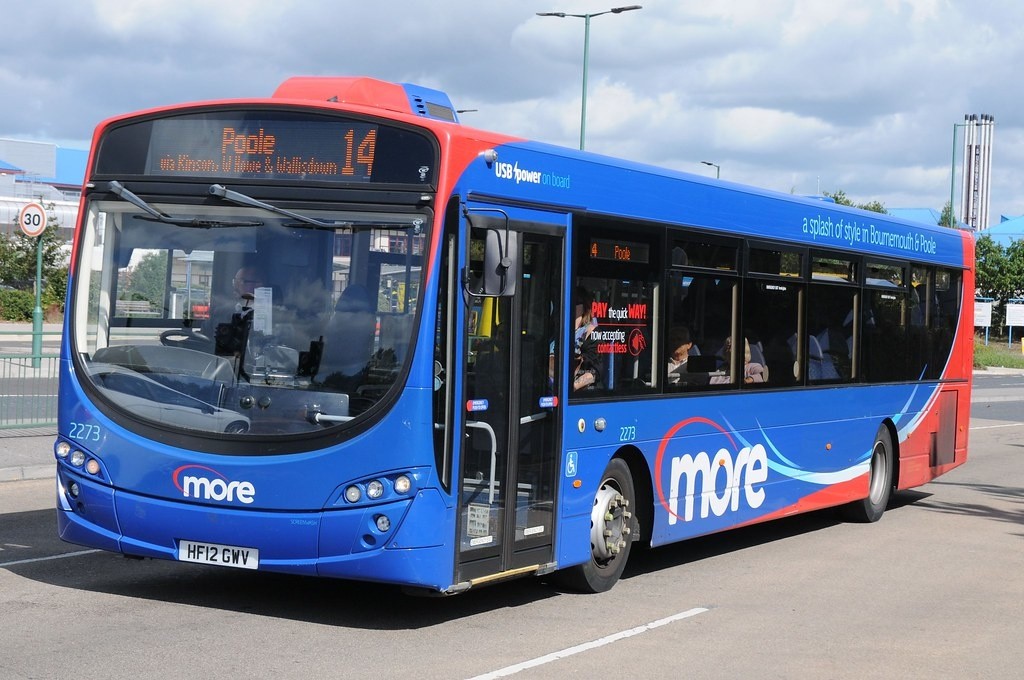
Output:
[19,201,47,238]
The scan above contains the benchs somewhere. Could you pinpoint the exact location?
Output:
[115,300,161,318]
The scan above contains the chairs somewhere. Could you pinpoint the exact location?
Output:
[688,284,940,380]
[315,283,376,385]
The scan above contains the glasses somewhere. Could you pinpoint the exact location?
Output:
[232,277,263,287]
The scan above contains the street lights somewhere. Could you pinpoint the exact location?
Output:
[534,4,645,152]
[949,122,983,227]
[700,160,720,180]
[240,291,255,307]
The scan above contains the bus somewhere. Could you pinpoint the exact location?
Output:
[52,75,977,599]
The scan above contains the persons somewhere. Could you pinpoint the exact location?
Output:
[185,260,270,356]
[639,277,764,392]
[549,287,604,390]
